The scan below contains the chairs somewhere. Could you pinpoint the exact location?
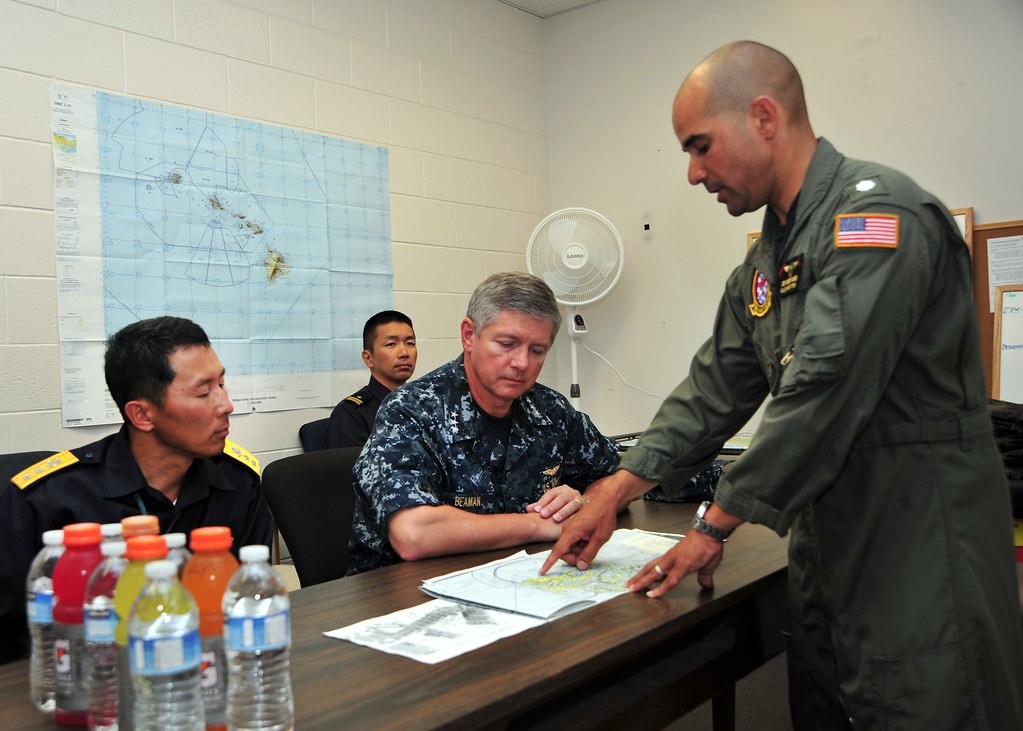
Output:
[0,420,365,588]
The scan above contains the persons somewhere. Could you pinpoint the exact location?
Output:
[328,310,417,449]
[540,38,1023,731]
[345,271,622,576]
[0,316,274,666]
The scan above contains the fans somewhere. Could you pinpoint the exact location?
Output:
[525,207,624,412]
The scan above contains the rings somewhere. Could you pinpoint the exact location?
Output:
[575,499,581,508]
[655,565,663,576]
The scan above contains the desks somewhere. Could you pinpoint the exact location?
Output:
[0,498,788,731]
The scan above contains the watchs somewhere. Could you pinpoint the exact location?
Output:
[692,501,730,542]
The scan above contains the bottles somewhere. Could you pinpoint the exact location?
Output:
[27,523,115,724]
[83,524,128,731]
[221,545,293,731]
[181,526,240,731]
[128,559,205,731]
[162,532,192,581]
[115,514,169,731]
